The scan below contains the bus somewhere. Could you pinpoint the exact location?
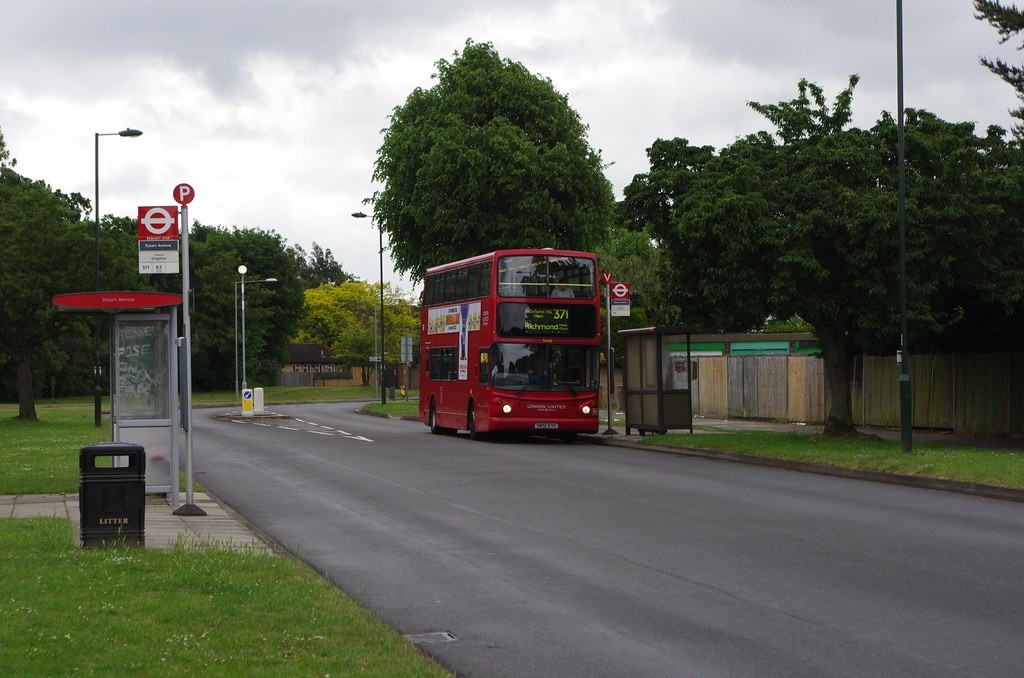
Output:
[417,246,602,441]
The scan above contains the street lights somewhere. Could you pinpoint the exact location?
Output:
[238,264,248,389]
[351,211,389,404]
[235,277,277,400]
[92,129,145,426]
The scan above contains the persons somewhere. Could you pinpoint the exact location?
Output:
[492,352,518,379]
[551,278,574,297]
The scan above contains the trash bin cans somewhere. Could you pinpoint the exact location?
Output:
[78,442,147,549]
